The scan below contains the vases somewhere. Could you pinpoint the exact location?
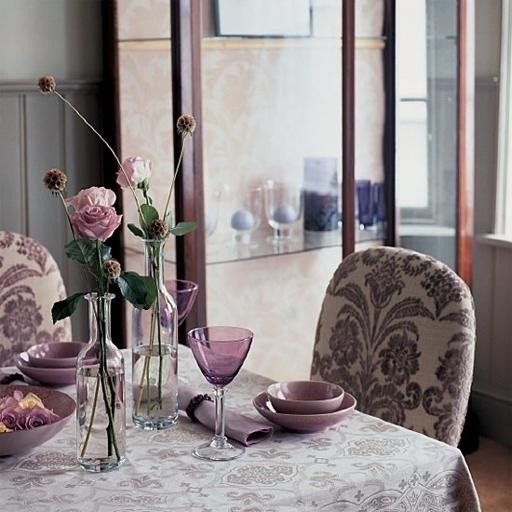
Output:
[125,233,181,431]
[301,155,341,234]
[74,292,128,475]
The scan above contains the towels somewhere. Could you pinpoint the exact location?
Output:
[175,379,274,448]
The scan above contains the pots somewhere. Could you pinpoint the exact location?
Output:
[1,385,77,456]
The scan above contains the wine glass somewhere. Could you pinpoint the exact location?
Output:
[263,186,302,245]
[158,279,199,339]
[204,189,224,252]
[186,325,250,459]
[228,190,266,251]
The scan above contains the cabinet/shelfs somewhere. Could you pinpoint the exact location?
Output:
[117,34,387,269]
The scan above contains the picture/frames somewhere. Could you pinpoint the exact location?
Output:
[210,1,316,39]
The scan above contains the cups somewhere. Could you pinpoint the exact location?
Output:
[305,157,340,232]
[360,178,387,236]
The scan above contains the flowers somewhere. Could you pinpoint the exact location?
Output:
[0,386,69,434]
[34,73,202,417]
[41,165,126,463]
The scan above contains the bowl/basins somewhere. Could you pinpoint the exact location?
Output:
[267,379,344,414]
[28,342,85,367]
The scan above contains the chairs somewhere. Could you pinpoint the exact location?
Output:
[306,244,480,447]
[0,226,78,370]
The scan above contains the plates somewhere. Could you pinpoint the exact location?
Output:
[18,350,100,385]
[252,385,358,435]
